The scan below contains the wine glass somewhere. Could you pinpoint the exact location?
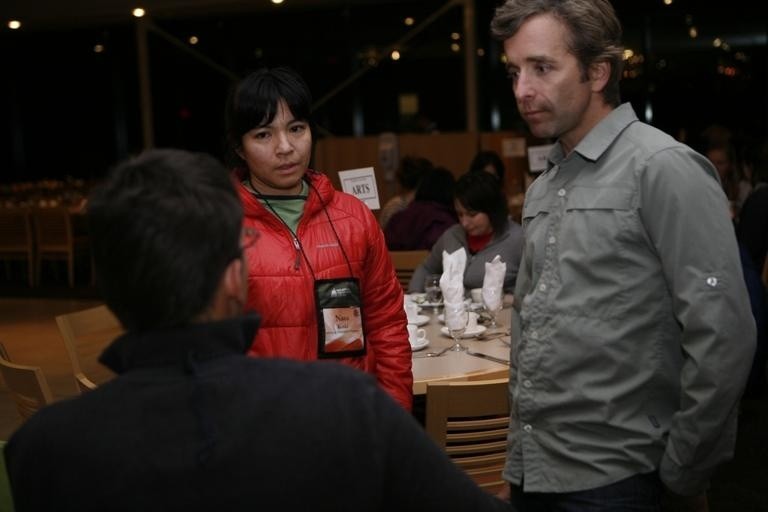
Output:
[444,304,469,352]
[480,286,504,330]
[424,273,443,321]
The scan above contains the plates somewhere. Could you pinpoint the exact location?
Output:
[437,312,480,323]
[405,315,431,326]
[407,337,429,350]
[440,326,486,339]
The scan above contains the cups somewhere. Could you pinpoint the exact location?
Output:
[470,288,483,304]
[406,324,426,345]
[404,303,421,321]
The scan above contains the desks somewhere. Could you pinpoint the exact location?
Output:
[410,296,514,397]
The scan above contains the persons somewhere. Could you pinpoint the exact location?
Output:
[409,170,526,296]
[703,133,766,251]
[488,1,757,511]
[378,133,507,253]
[228,67,413,414]
[2,145,522,512]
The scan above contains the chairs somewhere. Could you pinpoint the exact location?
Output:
[426,378,512,494]
[55,303,129,398]
[1,345,55,426]
[388,251,431,293]
[0,186,97,289]
